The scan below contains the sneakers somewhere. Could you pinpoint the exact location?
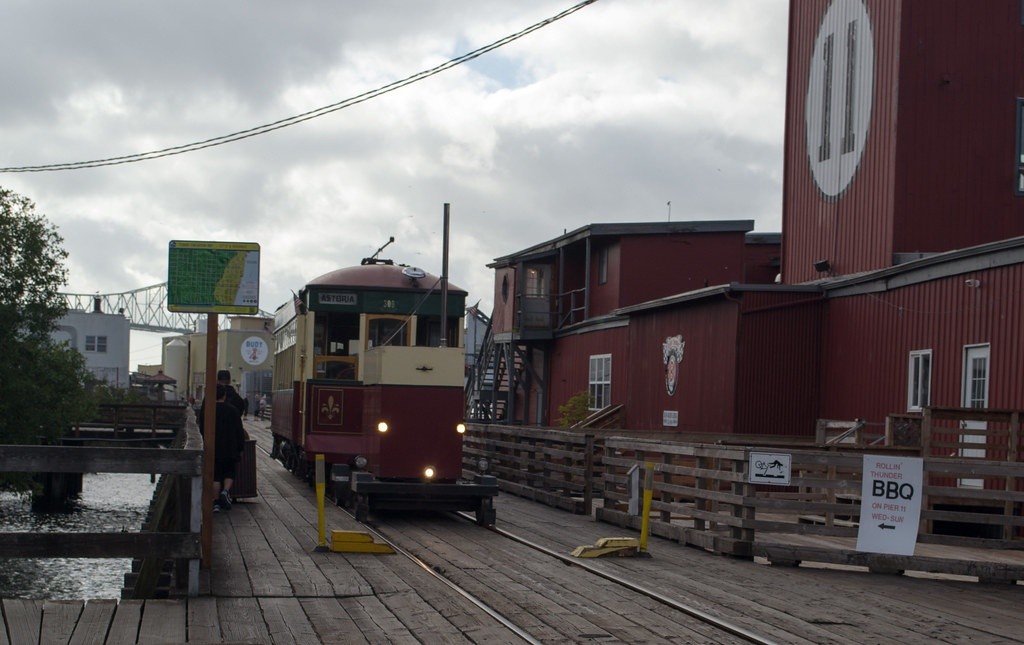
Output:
[212,489,233,513]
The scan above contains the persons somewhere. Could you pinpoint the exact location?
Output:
[189,394,195,408]
[377,327,400,346]
[181,393,185,405]
[198,382,246,513]
[199,369,246,418]
[242,394,249,421]
[259,394,266,421]
[253,394,260,421]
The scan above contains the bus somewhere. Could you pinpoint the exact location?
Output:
[268,264,500,527]
